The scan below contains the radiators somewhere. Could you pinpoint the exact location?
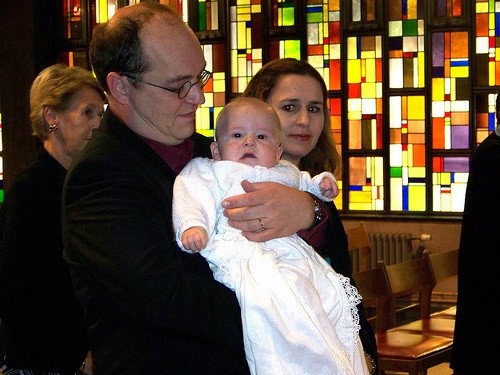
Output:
[348,232,432,297]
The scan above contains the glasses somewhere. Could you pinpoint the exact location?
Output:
[118,69,212,99]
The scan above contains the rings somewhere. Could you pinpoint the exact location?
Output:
[259,219,264,228]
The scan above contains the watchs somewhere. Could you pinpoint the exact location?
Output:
[309,200,322,231]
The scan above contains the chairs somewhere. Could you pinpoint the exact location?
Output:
[346,221,459,375]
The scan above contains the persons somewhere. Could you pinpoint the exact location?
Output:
[65,1,331,375]
[241,58,378,375]
[449,87,500,375]
[0,64,106,375]
[172,96,370,375]
